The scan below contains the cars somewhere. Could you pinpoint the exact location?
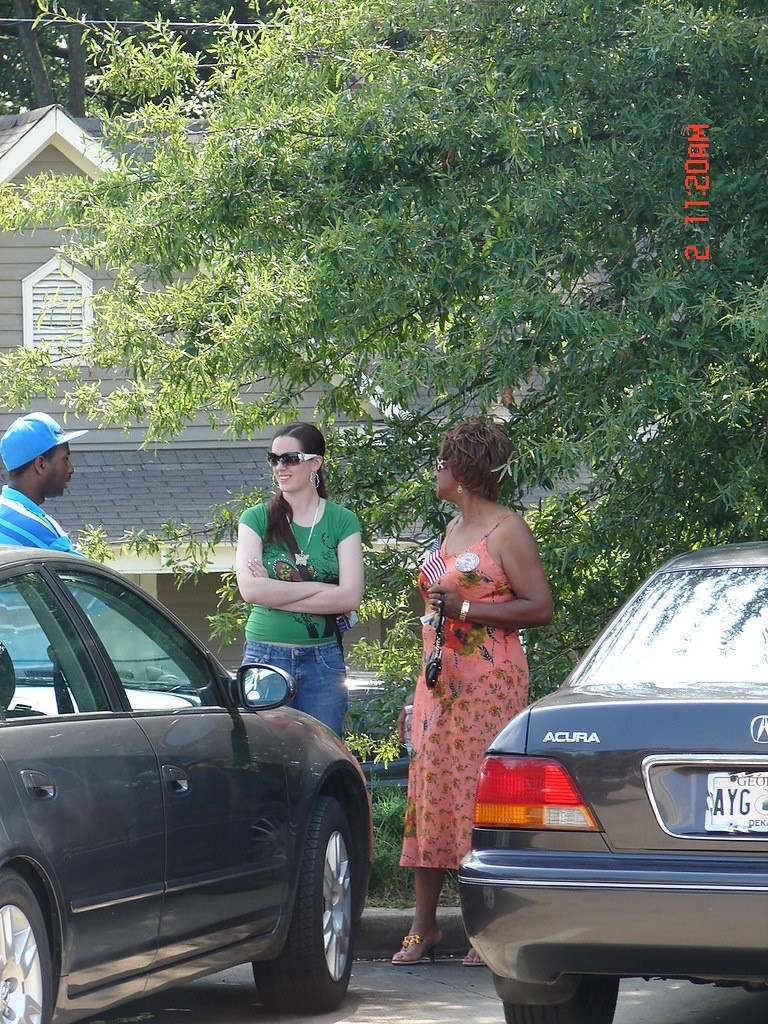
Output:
[454,543,768,1024]
[0,545,372,1024]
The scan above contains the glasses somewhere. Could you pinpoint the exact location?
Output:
[436,457,449,471]
[267,451,317,466]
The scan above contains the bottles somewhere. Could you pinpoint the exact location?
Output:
[404,705,412,756]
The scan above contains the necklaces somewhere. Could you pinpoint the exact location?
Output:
[287,498,320,566]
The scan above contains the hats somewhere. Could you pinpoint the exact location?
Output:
[0,411,88,472]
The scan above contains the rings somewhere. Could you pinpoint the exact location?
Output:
[439,608,440,612]
[438,600,441,604]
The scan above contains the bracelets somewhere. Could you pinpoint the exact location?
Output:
[458,599,471,622]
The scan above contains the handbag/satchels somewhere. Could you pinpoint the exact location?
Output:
[426,594,445,691]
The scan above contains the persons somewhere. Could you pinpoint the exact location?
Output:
[236,423,364,739]
[0,412,107,659]
[390,420,551,966]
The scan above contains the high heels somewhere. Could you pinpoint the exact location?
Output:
[462,960,487,966]
[391,935,442,966]
